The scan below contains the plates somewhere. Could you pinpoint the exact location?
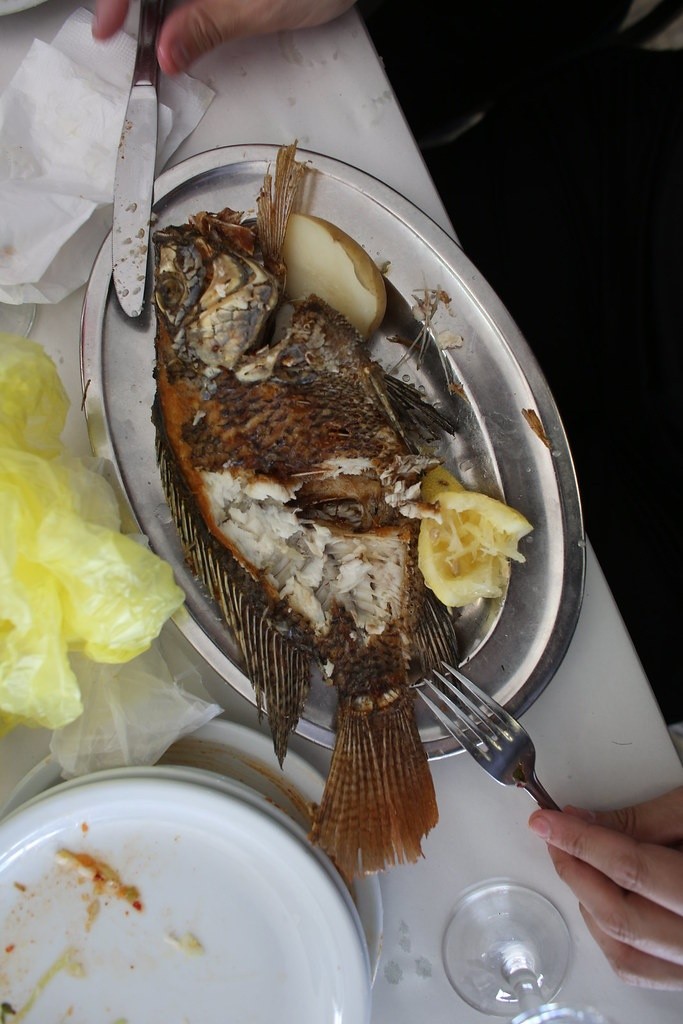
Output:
[0,722,385,1024]
[79,143,586,761]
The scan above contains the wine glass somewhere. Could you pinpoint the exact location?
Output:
[441,883,611,1024]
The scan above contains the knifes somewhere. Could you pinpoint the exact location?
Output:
[111,0,170,317]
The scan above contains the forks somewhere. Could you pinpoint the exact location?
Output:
[416,663,563,813]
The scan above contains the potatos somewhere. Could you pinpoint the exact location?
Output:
[279,214,387,343]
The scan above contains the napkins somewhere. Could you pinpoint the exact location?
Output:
[0,7,217,304]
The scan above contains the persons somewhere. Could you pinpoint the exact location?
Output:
[92,0,683,993]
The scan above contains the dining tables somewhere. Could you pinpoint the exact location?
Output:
[0,0,682,1019]
